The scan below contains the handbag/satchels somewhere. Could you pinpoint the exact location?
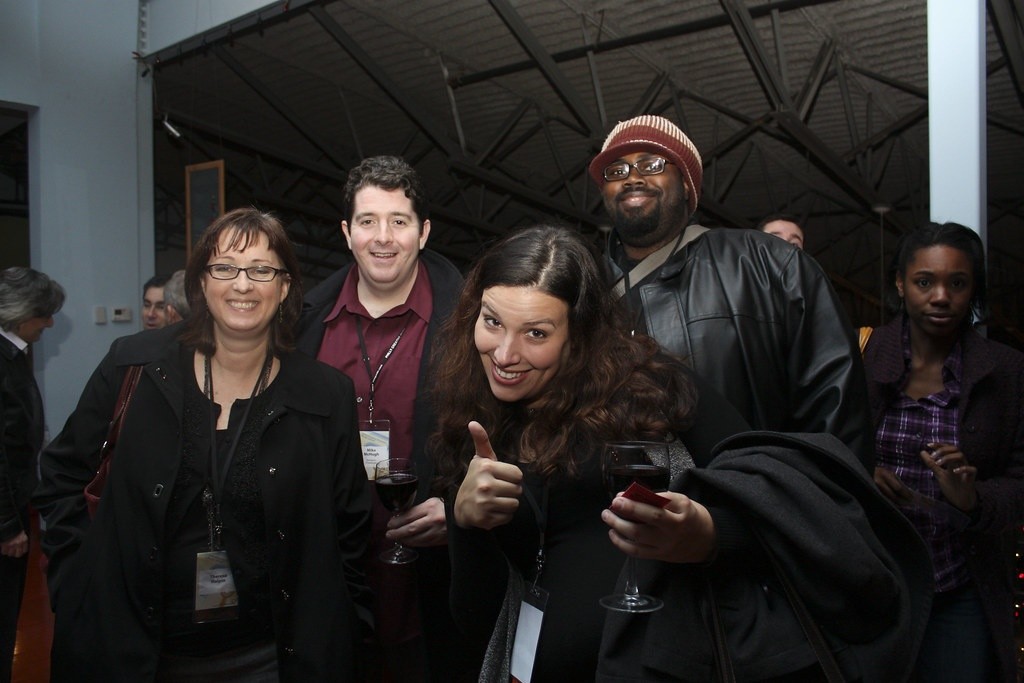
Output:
[83,365,145,527]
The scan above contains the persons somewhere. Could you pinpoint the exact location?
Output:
[133,272,166,328]
[0,265,70,680]
[280,153,477,681]
[587,111,874,477]
[845,220,1024,682]
[38,205,391,683]
[427,225,759,682]
[163,269,193,328]
[755,213,806,251]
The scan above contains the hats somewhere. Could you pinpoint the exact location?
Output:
[588,114,704,222]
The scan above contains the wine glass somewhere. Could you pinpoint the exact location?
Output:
[374,458,420,563]
[598,440,671,612]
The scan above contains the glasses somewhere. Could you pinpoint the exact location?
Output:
[204,263,288,282]
[602,157,676,181]
